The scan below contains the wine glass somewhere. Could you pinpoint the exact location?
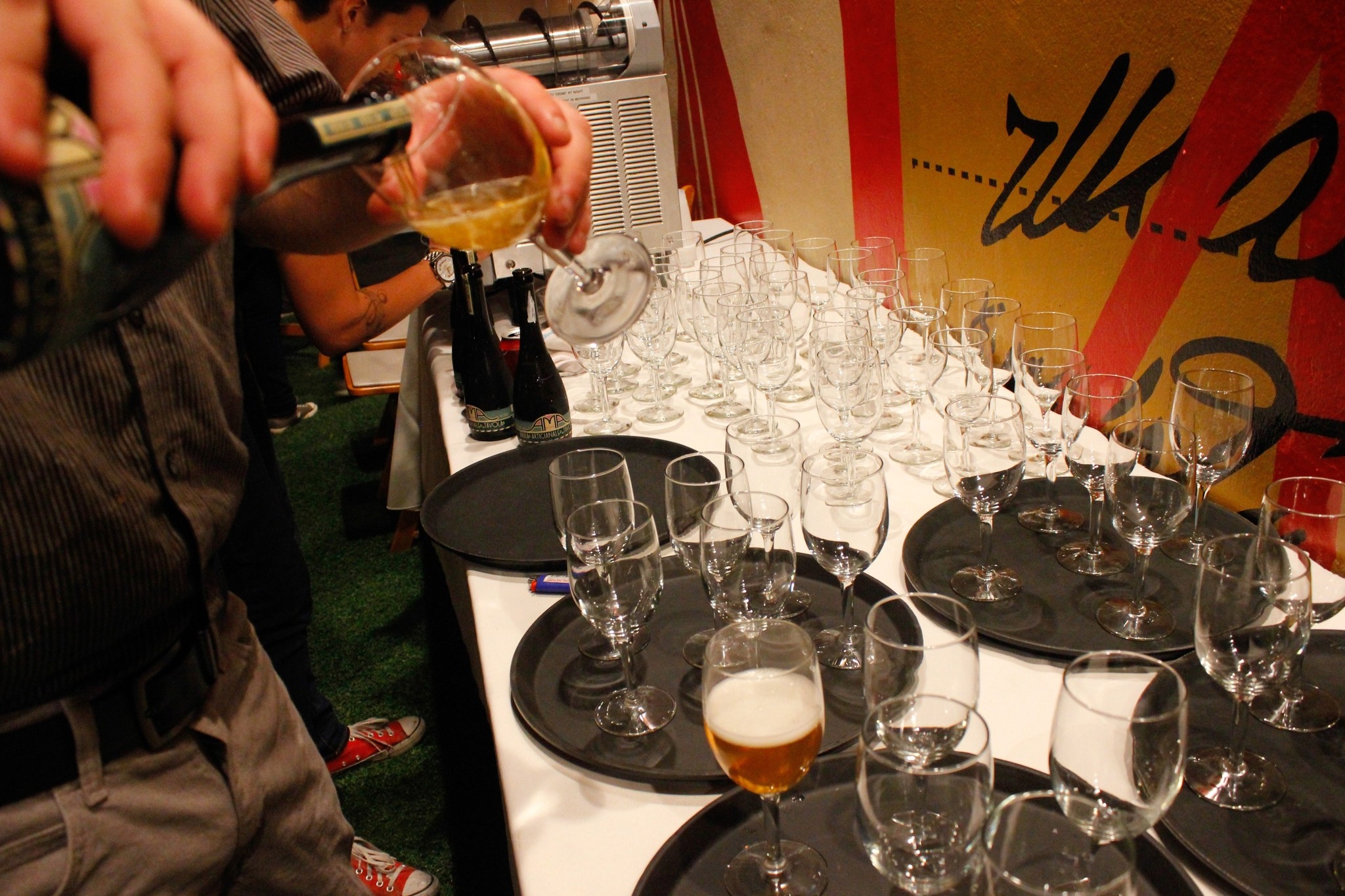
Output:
[1185,533,1312,812]
[702,620,829,896]
[1248,476,1345,733]
[1048,651,1188,865]
[863,592,980,854]
[537,215,1089,740]
[1158,367,1253,567]
[337,38,653,343]
[1058,373,1144,576]
[1095,420,1197,642]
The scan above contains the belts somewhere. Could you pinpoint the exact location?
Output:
[0,628,215,812]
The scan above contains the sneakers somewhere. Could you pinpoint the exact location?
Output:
[271,402,318,435]
[326,712,427,777]
[349,834,439,896]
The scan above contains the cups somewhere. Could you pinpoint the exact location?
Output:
[981,789,1139,895]
[853,695,994,896]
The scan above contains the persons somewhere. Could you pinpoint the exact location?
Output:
[0,0,590,896]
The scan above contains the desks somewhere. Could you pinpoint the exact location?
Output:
[382,211,1345,896]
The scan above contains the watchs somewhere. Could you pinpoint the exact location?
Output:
[422,252,455,288]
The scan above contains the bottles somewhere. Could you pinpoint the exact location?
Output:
[447,246,572,448]
[0,88,412,372]
